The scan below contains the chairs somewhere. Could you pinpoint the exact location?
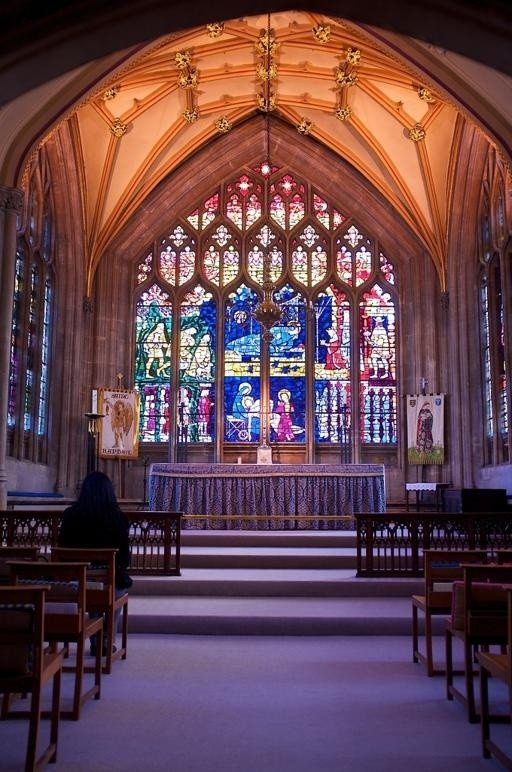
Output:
[1,535,129,771]
[405,545,511,772]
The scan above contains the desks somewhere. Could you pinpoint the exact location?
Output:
[142,461,387,531]
[402,481,453,512]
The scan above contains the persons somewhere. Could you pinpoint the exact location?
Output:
[57,469,131,657]
